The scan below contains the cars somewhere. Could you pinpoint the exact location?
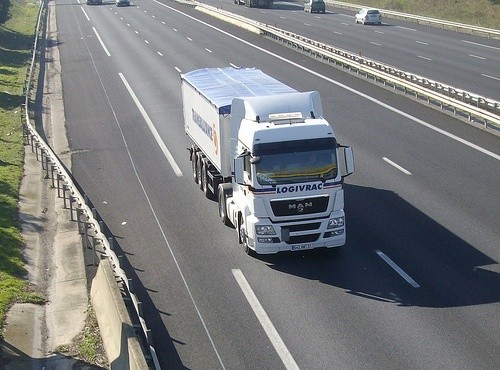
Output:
[116,0,131,7]
[86,0,102,5]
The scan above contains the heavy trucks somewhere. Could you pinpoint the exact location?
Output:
[180,66,355,256]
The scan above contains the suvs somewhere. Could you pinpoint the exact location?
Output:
[354,8,382,26]
[304,0,325,13]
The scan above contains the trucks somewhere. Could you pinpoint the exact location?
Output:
[233,0,273,7]
